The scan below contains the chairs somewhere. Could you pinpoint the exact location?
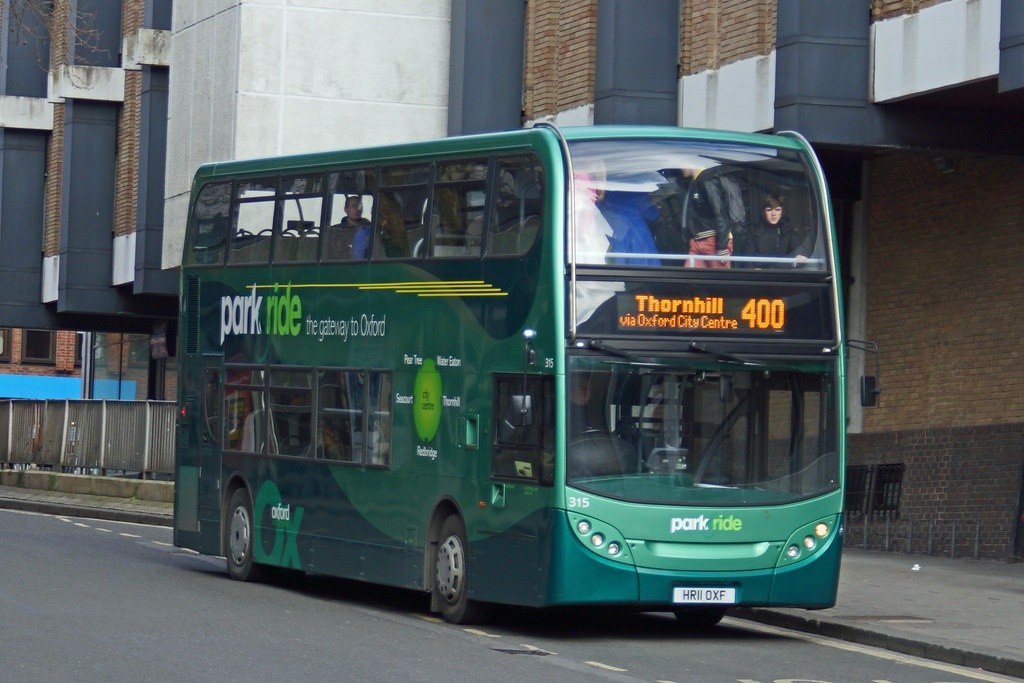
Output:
[275,384,356,458]
[228,212,540,259]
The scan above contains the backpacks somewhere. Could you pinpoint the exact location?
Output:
[721,174,745,232]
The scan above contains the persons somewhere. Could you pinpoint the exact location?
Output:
[734,195,812,269]
[679,156,746,269]
[330,196,373,261]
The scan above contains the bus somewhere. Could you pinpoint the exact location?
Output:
[169,118,850,631]
